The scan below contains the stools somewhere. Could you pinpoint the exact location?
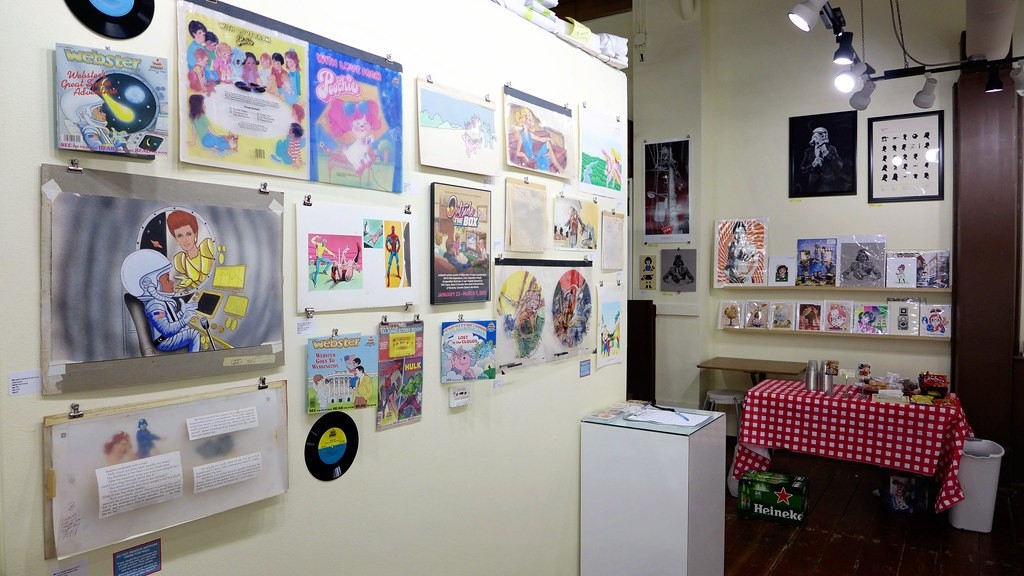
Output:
[702,390,746,412]
[705,397,744,444]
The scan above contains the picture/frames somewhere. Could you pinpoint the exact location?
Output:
[788,110,857,199]
[868,109,945,202]
[431,180,493,306]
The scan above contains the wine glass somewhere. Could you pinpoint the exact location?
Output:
[841,361,852,397]
[855,362,871,399]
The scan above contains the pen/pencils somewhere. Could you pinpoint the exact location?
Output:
[676,411,690,421]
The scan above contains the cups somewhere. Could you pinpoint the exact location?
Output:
[805,360,833,393]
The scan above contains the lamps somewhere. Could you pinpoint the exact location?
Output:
[786,0,829,32]
[833,62,867,93]
[849,79,878,111]
[913,71,938,108]
[984,62,1005,93]
[832,24,854,66]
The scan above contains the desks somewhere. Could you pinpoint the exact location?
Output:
[697,356,808,388]
[726,378,977,516]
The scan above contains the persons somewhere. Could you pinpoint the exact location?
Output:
[799,127,843,192]
[723,222,760,283]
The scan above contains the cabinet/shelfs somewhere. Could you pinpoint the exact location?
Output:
[721,283,955,342]
[580,401,723,576]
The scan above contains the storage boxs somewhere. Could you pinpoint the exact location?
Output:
[736,471,809,526]
[949,437,1006,534]
